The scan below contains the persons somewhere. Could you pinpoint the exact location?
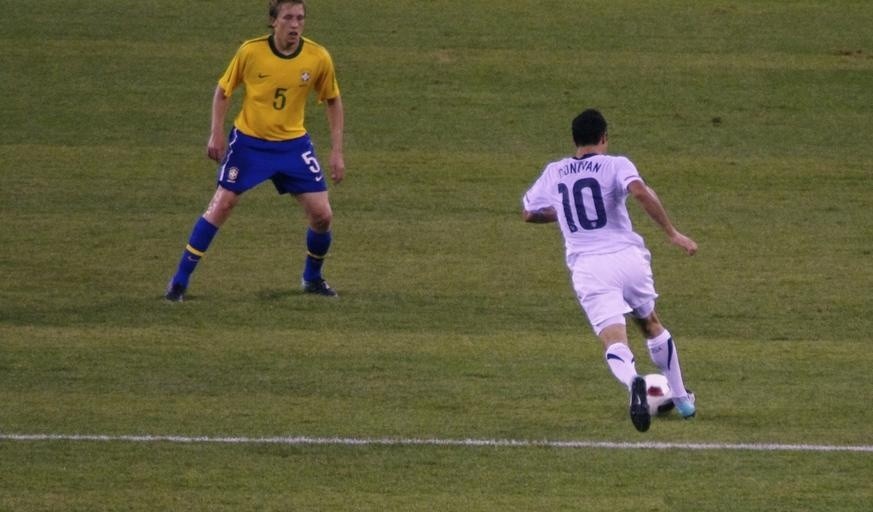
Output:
[522,106,700,435]
[161,1,347,305]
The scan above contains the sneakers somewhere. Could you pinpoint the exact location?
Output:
[628,375,650,431]
[163,276,186,304]
[671,388,696,420]
[301,278,337,298]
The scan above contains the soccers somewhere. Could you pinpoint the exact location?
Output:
[643,375,674,416]
